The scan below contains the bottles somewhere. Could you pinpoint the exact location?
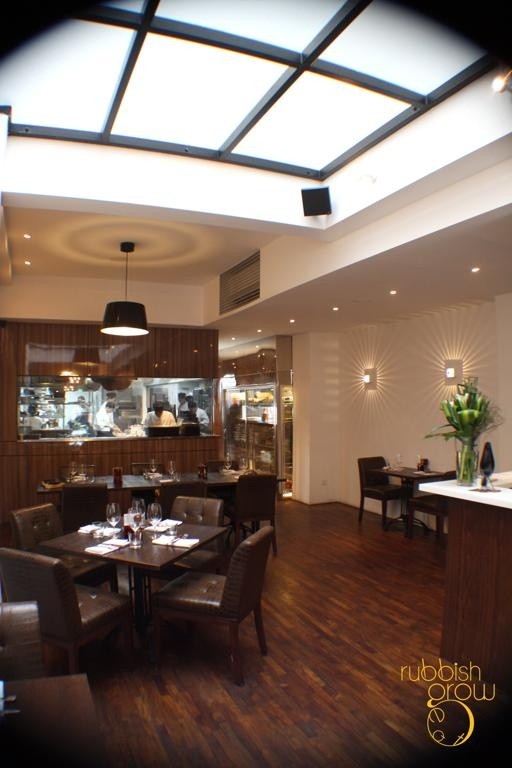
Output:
[262,407,268,423]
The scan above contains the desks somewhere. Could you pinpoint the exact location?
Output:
[0,672,107,767]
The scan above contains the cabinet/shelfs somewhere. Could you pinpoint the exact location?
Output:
[222,384,293,498]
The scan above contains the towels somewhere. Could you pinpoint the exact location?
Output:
[143,517,183,532]
[76,522,121,538]
[84,537,129,555]
[151,535,199,549]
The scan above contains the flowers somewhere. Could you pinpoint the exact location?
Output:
[422,378,508,484]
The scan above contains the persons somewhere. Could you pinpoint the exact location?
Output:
[22,391,211,437]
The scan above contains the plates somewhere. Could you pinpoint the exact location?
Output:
[232,425,247,441]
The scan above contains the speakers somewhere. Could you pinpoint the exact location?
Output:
[301,188,331,216]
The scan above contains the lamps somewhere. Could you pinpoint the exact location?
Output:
[72,347,101,365]
[492,74,512,93]
[99,239,149,338]
[444,358,463,385]
[362,368,378,390]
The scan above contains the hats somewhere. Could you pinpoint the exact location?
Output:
[188,399,196,408]
[153,401,164,411]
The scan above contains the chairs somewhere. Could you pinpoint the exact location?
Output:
[27,455,279,558]
[356,454,408,527]
[408,456,456,536]
[9,501,119,593]
[0,546,134,672]
[1,598,46,673]
[157,523,275,686]
[161,495,224,574]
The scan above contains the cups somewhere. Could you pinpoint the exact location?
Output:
[112,467,123,484]
[166,460,176,481]
[395,453,403,470]
[69,460,97,486]
[149,459,158,475]
[223,454,233,472]
[195,463,209,479]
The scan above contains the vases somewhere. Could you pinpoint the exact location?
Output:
[454,433,480,485]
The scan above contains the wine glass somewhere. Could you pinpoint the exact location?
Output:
[414,454,424,472]
[93,496,178,552]
